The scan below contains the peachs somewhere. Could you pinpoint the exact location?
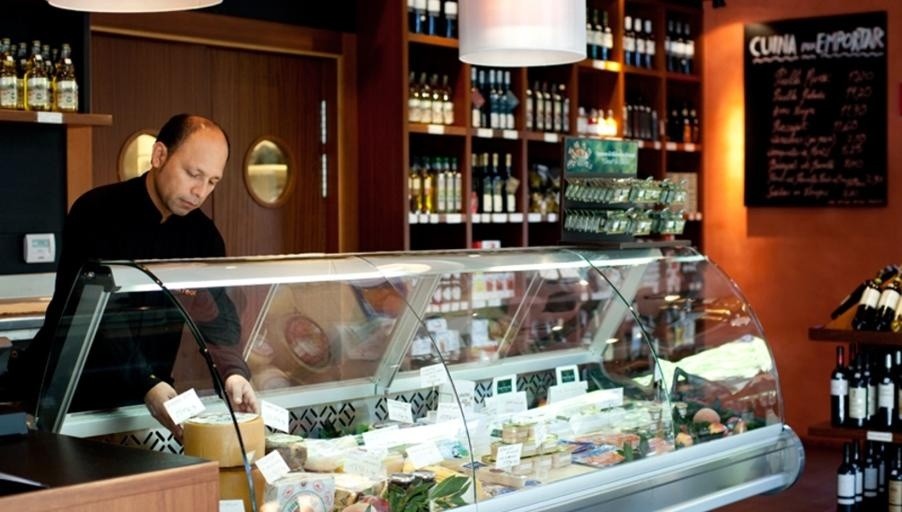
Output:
[675,407,746,447]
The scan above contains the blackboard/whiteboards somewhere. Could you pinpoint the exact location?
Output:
[744,10,888,208]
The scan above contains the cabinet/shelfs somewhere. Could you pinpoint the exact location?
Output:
[0,433,222,508]
[802,303,900,445]
[360,0,705,350]
[30,238,805,509]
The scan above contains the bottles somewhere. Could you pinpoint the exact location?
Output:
[0,33,79,113]
[407,153,462,214]
[407,0,459,40]
[666,107,699,144]
[471,151,516,214]
[623,15,656,70]
[826,263,902,510]
[622,100,659,142]
[526,74,572,133]
[577,106,618,138]
[586,6,613,62]
[470,62,520,129]
[408,63,457,125]
[666,16,695,75]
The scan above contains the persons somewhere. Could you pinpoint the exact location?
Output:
[7,114,259,447]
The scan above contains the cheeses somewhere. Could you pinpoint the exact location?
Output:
[183,411,265,469]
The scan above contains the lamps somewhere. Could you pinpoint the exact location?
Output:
[49,1,225,14]
[457,1,587,68]
